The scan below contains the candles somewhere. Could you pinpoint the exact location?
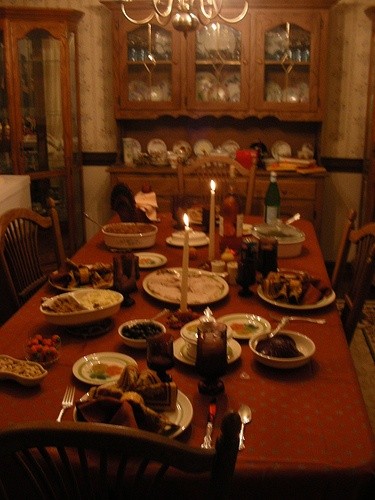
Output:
[210,180,216,257]
[180,213,189,310]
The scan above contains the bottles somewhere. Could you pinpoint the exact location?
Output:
[263,171,280,223]
[211,253,237,286]
[216,181,244,254]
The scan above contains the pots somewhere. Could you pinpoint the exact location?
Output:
[170,335,242,369]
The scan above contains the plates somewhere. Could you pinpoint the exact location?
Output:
[122,19,310,104]
[255,281,336,310]
[217,313,273,340]
[166,236,209,247]
[142,266,229,306]
[38,252,194,440]
[120,138,317,166]
[248,332,316,367]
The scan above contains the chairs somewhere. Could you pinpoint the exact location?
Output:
[0,411,242,500]
[0,197,64,309]
[331,208,375,313]
[177,152,257,217]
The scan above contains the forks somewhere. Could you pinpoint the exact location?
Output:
[55,384,75,424]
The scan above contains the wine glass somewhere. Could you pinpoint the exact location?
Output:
[236,239,279,298]
[194,322,229,396]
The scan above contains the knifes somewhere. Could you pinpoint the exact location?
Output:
[200,398,218,450]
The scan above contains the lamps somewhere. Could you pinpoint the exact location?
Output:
[120,0,248,39]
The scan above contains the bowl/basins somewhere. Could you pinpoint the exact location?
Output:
[102,222,158,247]
[181,315,232,358]
[253,223,306,258]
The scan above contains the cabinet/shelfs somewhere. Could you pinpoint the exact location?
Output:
[98,0,339,219]
[0,7,85,263]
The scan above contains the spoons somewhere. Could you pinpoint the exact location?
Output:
[235,403,253,451]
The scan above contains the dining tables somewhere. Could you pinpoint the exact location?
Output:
[0,215,375,500]
